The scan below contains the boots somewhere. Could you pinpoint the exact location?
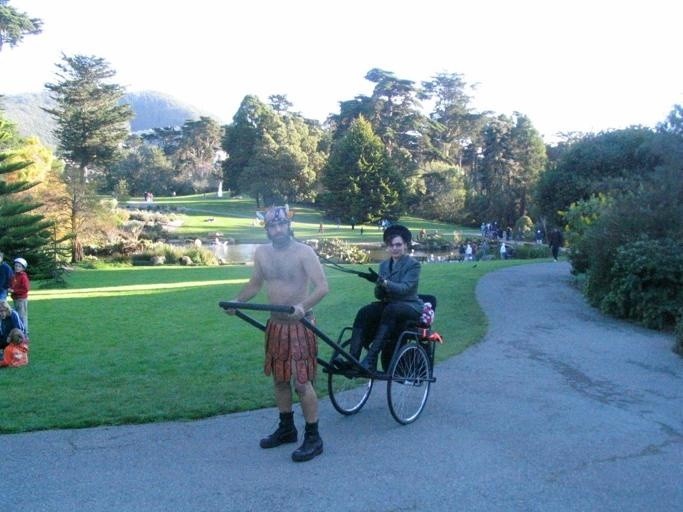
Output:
[365,325,392,372]
[260,412,297,449]
[294,418,324,462]
[335,326,365,368]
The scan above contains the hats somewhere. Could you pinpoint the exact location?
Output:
[383,225,412,243]
[263,205,294,226]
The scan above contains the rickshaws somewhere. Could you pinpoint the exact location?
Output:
[219,294,443,426]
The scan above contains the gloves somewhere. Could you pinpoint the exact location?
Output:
[357,266,377,282]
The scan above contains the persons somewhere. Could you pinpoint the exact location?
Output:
[224,205,328,461]
[335,225,424,379]
[0,253,30,367]
[144,192,154,202]
[416,221,562,262]
[319,217,390,236]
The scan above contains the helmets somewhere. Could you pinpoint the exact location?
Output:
[15,258,27,272]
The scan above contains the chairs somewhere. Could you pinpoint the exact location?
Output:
[368,295,436,348]
